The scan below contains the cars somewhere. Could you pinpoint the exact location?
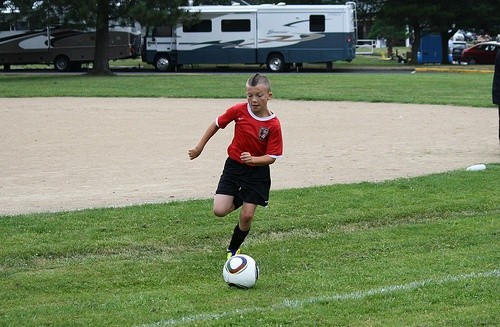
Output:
[461,41,499,66]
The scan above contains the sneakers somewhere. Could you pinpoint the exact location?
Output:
[226,248,240,260]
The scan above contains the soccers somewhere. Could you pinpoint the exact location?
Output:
[223,254,259,289]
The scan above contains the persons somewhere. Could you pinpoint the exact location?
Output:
[491,43,500,140]
[188,72,283,260]
[387,43,393,58]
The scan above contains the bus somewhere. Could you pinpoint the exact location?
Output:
[140,3,354,72]
[0,9,139,71]
